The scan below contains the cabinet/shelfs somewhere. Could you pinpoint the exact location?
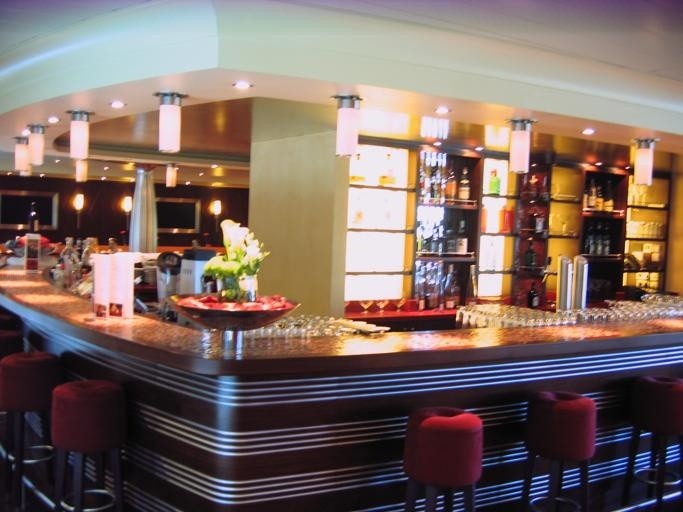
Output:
[343,137,671,308]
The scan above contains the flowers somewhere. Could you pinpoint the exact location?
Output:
[202,219,270,296]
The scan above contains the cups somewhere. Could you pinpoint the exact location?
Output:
[242,314,390,338]
[454,293,682,330]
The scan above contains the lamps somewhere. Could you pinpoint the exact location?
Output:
[334,95,363,158]
[153,89,188,154]
[26,123,47,167]
[72,159,89,184]
[166,163,179,187]
[65,109,94,160]
[13,135,29,171]
[505,118,536,173]
[633,137,659,188]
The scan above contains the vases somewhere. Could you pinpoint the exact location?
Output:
[238,273,259,301]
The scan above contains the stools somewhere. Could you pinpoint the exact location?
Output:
[621,374,682,502]
[50,381,124,511]
[2,353,58,491]
[406,406,483,511]
[520,392,597,511]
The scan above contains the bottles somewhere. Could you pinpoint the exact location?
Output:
[527,174,548,199]
[525,237,536,271]
[583,221,610,256]
[583,175,615,212]
[464,264,477,307]
[419,151,470,206]
[527,281,539,308]
[415,259,461,310]
[417,218,467,254]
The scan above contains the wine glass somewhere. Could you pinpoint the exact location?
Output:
[359,298,407,312]
